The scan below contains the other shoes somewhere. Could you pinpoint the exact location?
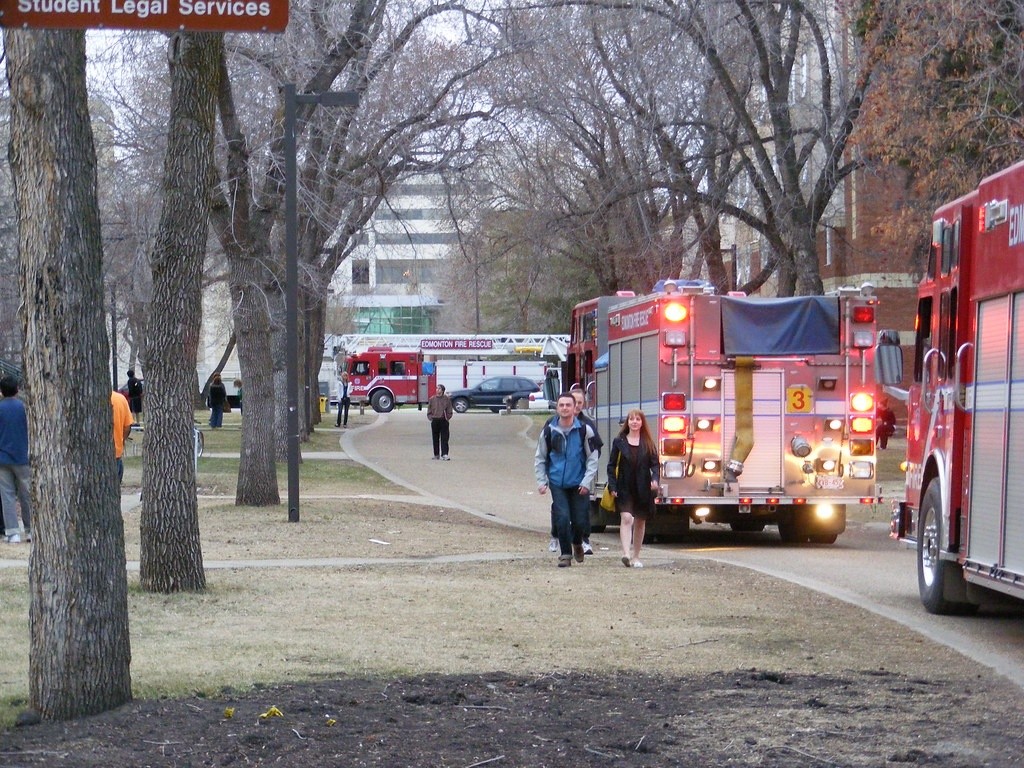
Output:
[432,455,440,459]
[25,534,31,541]
[622,556,631,567]
[632,561,643,568]
[131,423,141,427]
[442,454,450,460]
[335,423,340,427]
[3,534,20,542]
[343,424,346,428]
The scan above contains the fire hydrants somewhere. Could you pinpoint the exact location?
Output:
[319,392,329,414]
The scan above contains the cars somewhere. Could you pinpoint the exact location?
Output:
[119,377,144,396]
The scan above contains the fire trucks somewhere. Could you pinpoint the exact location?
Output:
[333,334,572,413]
[874,156,1023,615]
[543,278,885,544]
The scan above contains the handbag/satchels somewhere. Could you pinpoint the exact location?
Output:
[600,484,616,513]
[223,402,231,413]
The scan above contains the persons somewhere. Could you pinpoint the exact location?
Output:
[0,375,32,542]
[335,370,354,429]
[427,384,453,461]
[207,372,227,428]
[361,362,369,374]
[874,396,896,450]
[110,389,135,484]
[127,370,143,424]
[233,379,243,415]
[607,409,660,567]
[534,386,603,565]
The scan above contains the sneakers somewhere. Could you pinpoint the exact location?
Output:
[548,537,558,552]
[558,559,571,567]
[574,546,584,562]
[582,543,593,554]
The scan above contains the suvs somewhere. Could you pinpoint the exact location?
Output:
[448,376,540,413]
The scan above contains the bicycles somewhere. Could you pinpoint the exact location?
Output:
[194,419,205,458]
